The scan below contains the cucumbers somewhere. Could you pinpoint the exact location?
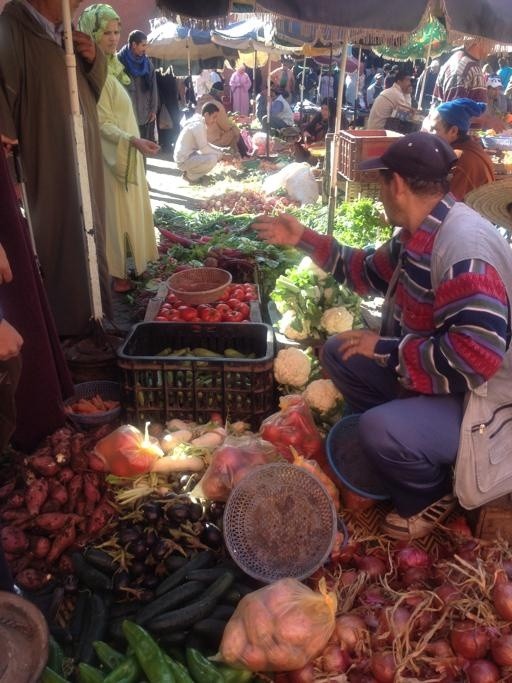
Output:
[36,549,247,669]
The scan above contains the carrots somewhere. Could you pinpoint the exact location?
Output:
[64,393,120,415]
[157,226,193,248]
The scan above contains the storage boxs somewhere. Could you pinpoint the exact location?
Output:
[118,321,275,432]
[155,279,261,303]
[145,297,262,322]
[338,130,404,184]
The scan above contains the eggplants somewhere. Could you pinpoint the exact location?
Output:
[93,500,224,601]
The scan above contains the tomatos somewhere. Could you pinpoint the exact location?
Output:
[155,282,258,323]
[259,400,327,469]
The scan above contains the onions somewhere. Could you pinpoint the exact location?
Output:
[290,515,512,683]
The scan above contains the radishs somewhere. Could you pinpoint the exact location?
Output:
[191,419,229,447]
[161,429,192,451]
[165,418,189,432]
[150,455,204,473]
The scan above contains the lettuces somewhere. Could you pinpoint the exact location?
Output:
[332,196,393,248]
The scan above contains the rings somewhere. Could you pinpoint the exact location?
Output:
[349,338,354,346]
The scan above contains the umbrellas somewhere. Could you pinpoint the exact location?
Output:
[157,0,512,236]
[211,12,402,103]
[146,16,213,88]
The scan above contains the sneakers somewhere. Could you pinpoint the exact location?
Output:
[381,489,457,541]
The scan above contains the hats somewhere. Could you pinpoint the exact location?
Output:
[351,133,459,181]
[436,97,488,132]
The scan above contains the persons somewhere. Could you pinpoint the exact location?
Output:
[118,29,158,191]
[482,55,512,113]
[367,71,414,131]
[76,4,160,293]
[250,133,512,543]
[0,242,23,487]
[421,37,509,134]
[173,102,233,185]
[428,97,495,204]
[195,82,249,160]
[229,65,251,117]
[302,96,335,143]
[0,0,108,338]
[367,64,399,110]
[256,87,293,130]
[271,59,296,104]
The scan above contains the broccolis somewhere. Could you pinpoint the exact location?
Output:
[301,378,344,421]
[273,346,312,387]
[270,255,361,342]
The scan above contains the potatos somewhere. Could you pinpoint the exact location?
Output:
[0,420,115,592]
[220,581,315,673]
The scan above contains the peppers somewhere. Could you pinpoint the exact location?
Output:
[40,619,252,683]
[135,345,257,408]
